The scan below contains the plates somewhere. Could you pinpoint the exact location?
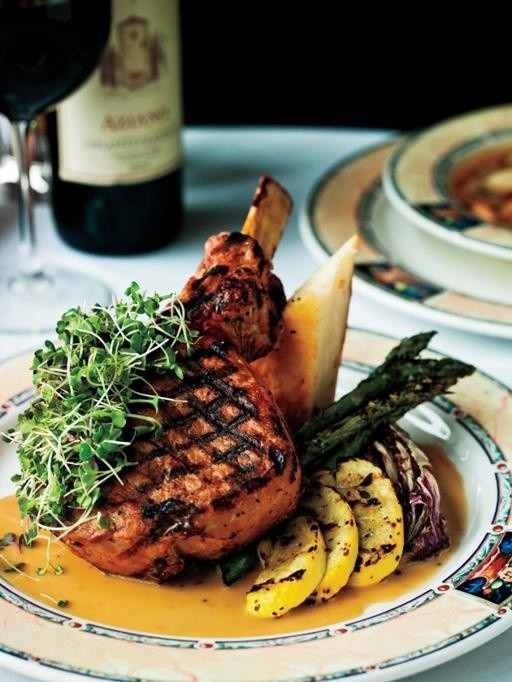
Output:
[298,138,510,341]
[385,103,511,264]
[1,323,511,682]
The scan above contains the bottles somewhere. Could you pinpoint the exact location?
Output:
[44,0,185,256]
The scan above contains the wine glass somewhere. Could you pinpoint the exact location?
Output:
[0,0,117,330]
